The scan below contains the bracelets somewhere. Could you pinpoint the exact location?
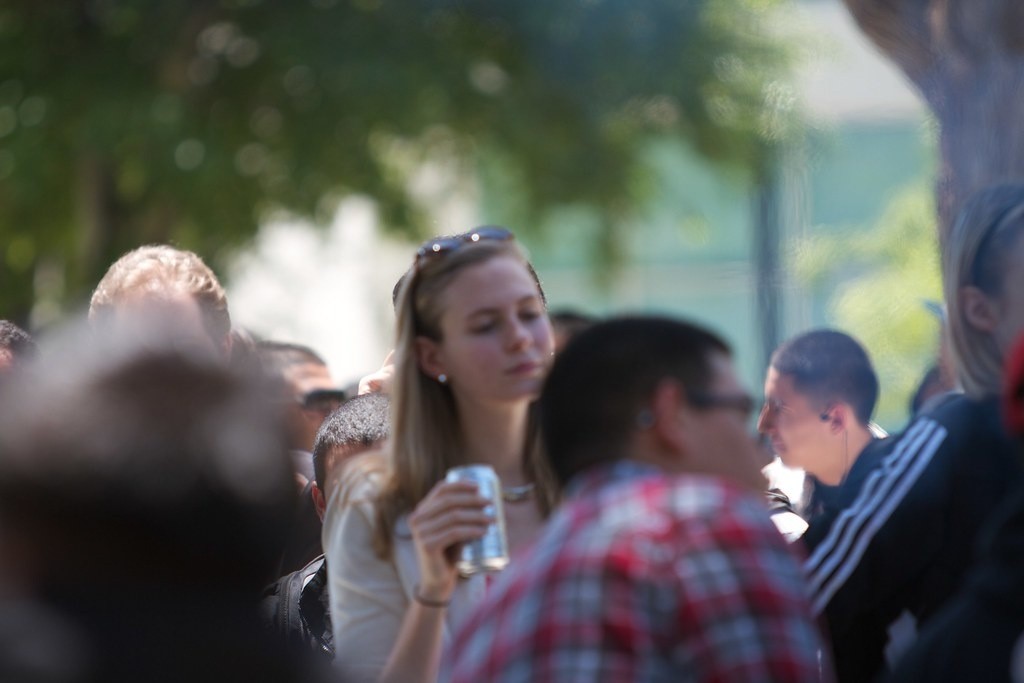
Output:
[411,582,452,610]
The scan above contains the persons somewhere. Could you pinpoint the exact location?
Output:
[263,392,391,663]
[87,246,233,361]
[0,320,45,404]
[358,349,396,395]
[256,341,338,455]
[445,317,831,683]
[798,178,1024,683]
[319,236,554,683]
[0,344,290,683]
[757,330,890,526]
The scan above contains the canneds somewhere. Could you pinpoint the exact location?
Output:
[444,463,509,574]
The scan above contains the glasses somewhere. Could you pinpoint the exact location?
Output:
[412,224,520,271]
[650,381,754,421]
